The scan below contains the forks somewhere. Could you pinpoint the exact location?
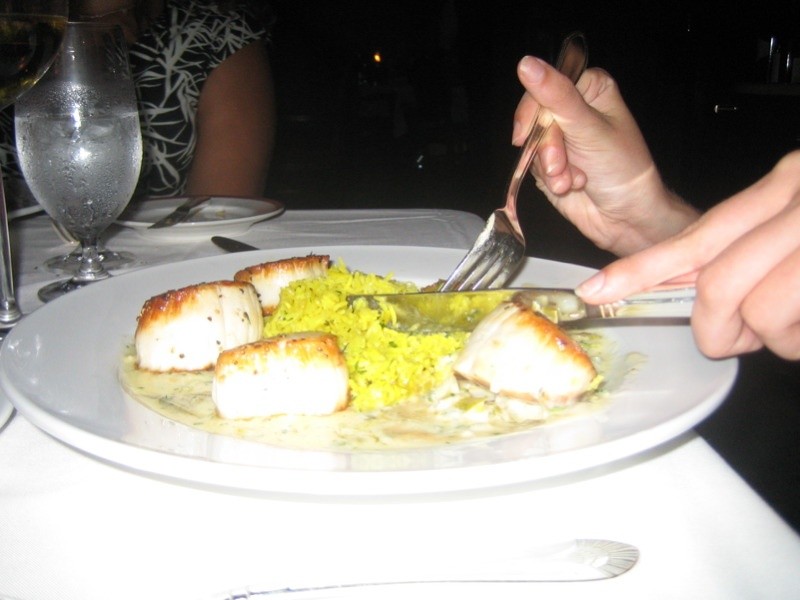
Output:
[439,33,591,291]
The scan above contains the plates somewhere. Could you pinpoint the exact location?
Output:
[1,240,742,497]
[110,192,283,240]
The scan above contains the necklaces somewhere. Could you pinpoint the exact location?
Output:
[74,3,139,22]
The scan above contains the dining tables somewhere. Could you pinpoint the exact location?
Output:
[1,207,800,600]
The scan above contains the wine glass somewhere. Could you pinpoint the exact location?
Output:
[2,1,72,338]
[14,18,142,308]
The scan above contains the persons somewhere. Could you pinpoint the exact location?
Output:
[511,54,799,361]
[0,0,289,212]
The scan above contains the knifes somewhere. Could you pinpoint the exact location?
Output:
[147,191,215,230]
[350,283,695,333]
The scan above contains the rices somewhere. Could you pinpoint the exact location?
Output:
[262,256,474,411]
